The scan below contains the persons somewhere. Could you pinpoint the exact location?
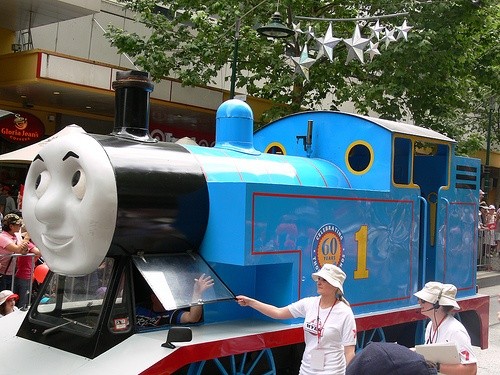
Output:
[134,273,214,328]
[478,190,500,270]
[0,133,128,316]
[234,263,356,375]
[413,281,477,375]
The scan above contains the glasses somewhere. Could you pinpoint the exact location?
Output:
[418,298,428,304]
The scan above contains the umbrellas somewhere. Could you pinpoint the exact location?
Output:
[0,124,86,165]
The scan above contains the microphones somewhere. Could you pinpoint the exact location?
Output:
[415,309,434,315]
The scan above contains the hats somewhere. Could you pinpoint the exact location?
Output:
[414,282,461,311]
[346,341,437,375]
[2,213,22,225]
[0,290,19,306]
[312,263,347,295]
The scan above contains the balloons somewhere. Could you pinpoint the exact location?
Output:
[34,264,49,283]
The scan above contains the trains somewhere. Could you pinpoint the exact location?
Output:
[0,69,491,375]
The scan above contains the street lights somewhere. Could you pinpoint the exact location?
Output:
[229,0,296,102]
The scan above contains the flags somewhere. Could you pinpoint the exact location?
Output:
[487,223,496,230]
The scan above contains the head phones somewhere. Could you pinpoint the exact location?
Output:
[432,287,444,309]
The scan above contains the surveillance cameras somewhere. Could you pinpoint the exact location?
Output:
[12,44,22,53]
